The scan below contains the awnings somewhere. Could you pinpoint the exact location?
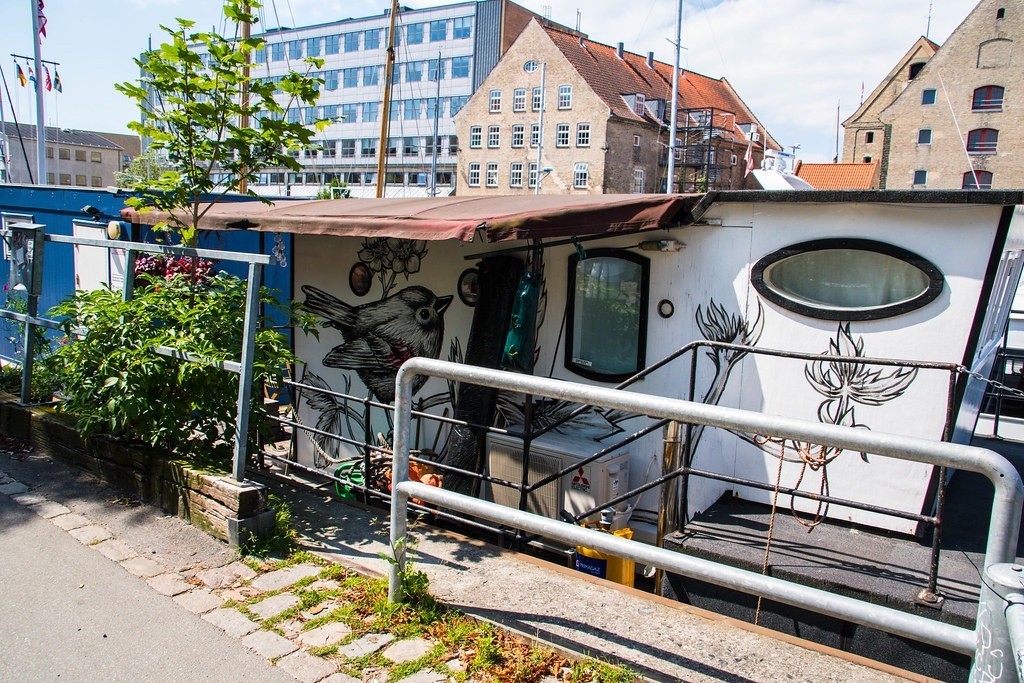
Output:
[121,194,706,243]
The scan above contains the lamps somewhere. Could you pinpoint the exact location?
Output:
[81,205,102,222]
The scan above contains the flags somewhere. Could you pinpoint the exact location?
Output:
[28,66,38,92]
[16,63,27,88]
[43,65,51,92]
[53,69,62,93]
[37,0,47,45]
[744,142,754,178]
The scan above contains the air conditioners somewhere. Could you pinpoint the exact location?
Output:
[485,424,629,548]
